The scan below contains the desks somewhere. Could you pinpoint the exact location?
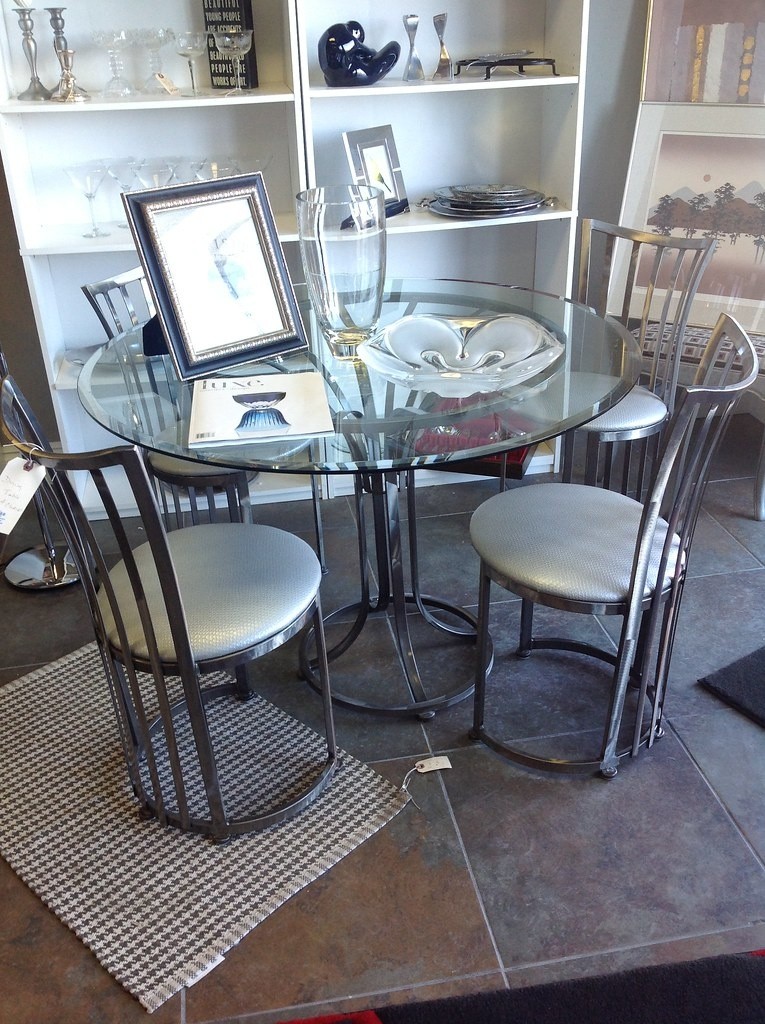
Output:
[77,279,641,721]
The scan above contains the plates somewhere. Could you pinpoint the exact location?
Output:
[429,184,544,218]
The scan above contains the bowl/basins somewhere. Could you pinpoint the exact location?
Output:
[356,313,565,398]
[63,343,164,371]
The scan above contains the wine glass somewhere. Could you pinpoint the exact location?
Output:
[64,163,111,238]
[91,29,138,100]
[174,31,210,97]
[100,156,147,228]
[135,28,174,96]
[213,30,254,96]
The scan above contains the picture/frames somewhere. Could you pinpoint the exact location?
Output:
[342,124,408,217]
[124,172,311,383]
[638,1,765,101]
[608,101,765,335]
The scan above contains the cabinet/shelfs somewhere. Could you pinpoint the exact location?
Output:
[1,4,591,530]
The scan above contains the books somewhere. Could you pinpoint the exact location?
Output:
[188,371,335,451]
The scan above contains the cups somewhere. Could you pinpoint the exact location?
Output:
[227,155,273,176]
[296,184,385,360]
[128,154,237,189]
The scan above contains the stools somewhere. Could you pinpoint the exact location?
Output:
[595,321,764,523]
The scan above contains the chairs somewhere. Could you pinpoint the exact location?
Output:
[501,221,711,506]
[469,314,759,779]
[0,379,333,833]
[81,264,258,527]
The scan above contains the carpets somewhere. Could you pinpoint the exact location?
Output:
[0,639,413,1013]
